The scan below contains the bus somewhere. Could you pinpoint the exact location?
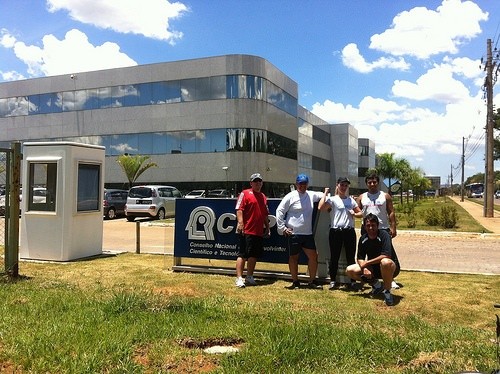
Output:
[465,183,485,198]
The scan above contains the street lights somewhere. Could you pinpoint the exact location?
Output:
[222,166,229,198]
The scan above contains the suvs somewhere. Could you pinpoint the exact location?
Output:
[124,185,184,222]
[104,188,130,220]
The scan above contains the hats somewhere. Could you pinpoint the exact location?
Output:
[250,173,263,182]
[336,176,351,184]
[296,173,309,184]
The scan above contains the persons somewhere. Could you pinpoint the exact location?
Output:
[346,213,400,306]
[355,173,400,288]
[235,172,270,288]
[276,174,331,289]
[318,177,364,291]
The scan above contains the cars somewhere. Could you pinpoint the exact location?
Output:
[183,190,208,199]
[208,190,227,198]
[0,187,47,216]
[495,190,500,198]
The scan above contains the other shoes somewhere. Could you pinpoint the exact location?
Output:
[383,289,395,306]
[350,282,361,291]
[390,280,400,290]
[285,280,301,289]
[368,283,385,298]
[328,280,338,290]
[308,280,323,289]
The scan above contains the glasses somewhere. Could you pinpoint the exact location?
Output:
[253,178,263,182]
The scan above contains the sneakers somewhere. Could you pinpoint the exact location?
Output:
[235,277,246,287]
[245,275,258,286]
